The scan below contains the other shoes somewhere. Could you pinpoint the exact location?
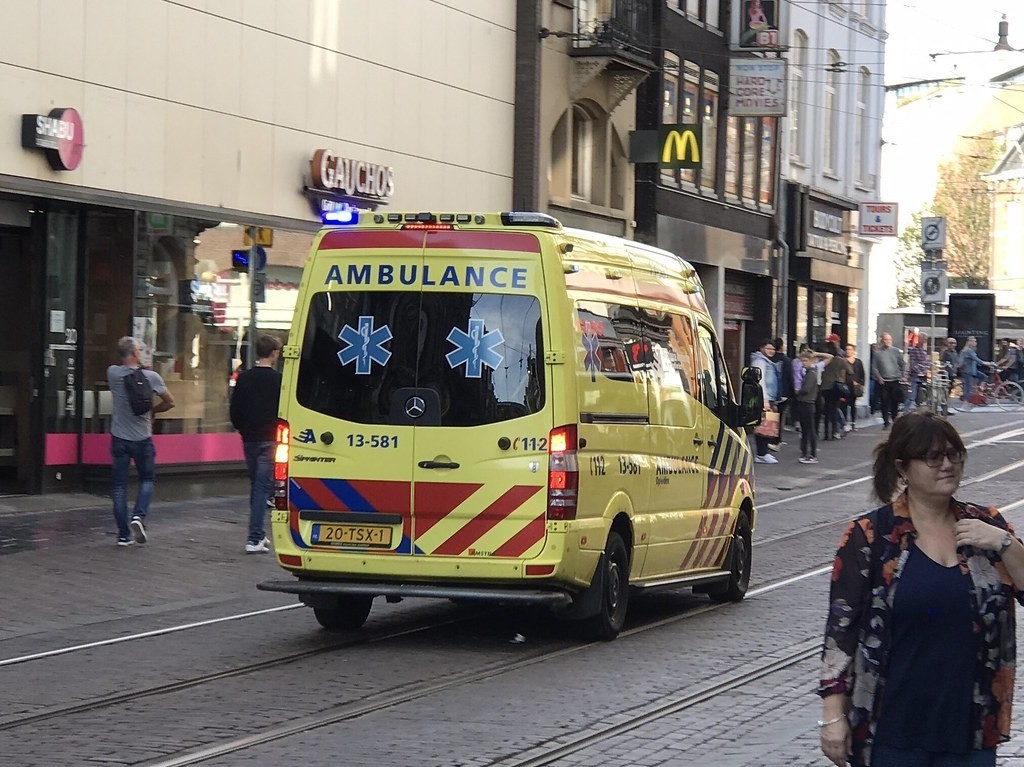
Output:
[260,536,270,546]
[833,433,841,439]
[245,540,269,553]
[882,421,891,431]
[117,536,136,547]
[794,422,801,432]
[754,454,778,464]
[783,426,795,432]
[798,456,818,464]
[130,517,147,544]
[266,499,275,507]
[768,443,780,452]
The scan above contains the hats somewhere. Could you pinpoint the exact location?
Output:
[824,334,841,341]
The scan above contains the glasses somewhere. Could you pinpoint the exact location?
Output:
[900,448,965,467]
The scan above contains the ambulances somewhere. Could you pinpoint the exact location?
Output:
[254,209,758,642]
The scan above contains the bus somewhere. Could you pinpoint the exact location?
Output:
[874,309,1024,358]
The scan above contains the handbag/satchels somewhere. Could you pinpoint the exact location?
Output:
[834,380,851,397]
[854,382,864,397]
[753,408,780,438]
[124,367,153,416]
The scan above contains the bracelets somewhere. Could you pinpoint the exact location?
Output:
[999,531,1011,554]
[818,714,845,726]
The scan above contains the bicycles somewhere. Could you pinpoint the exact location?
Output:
[948,366,1024,413]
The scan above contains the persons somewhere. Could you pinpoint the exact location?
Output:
[995,337,1024,396]
[794,353,818,463]
[960,336,997,401]
[750,339,778,464]
[767,338,794,452]
[816,410,1024,767]
[872,333,905,429]
[870,343,877,413]
[229,333,282,553]
[910,332,927,406]
[939,338,959,416]
[791,333,865,440]
[108,336,174,546]
